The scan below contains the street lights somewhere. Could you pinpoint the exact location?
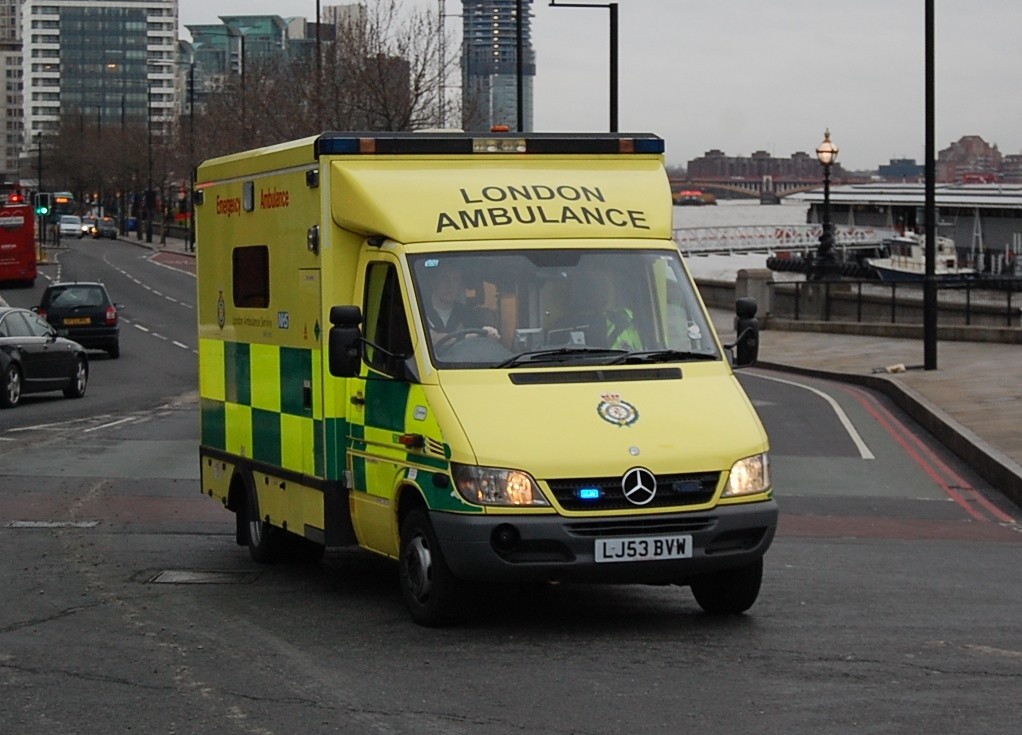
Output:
[549,1,620,134]
[274,14,297,51]
[225,24,257,151]
[814,128,839,276]
[35,130,44,194]
[72,83,156,244]
[179,39,209,253]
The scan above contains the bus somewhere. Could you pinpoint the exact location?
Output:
[51,192,75,225]
[0,182,37,285]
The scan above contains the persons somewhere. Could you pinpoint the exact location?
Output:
[421,260,501,356]
[548,266,642,351]
[1008,249,1017,274]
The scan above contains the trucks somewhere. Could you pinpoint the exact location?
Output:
[193,131,780,611]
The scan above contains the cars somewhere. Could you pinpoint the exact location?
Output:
[0,307,91,403]
[58,214,83,238]
[39,282,122,359]
[83,216,117,239]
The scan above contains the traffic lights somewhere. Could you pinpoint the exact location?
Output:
[40,194,47,215]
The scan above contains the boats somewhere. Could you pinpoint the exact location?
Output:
[866,233,974,289]
